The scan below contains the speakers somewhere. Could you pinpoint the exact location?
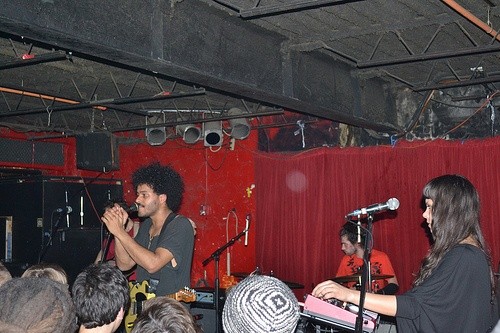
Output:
[75,132,119,171]
[22,185,124,283]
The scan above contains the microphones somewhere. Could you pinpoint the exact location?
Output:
[56,206,72,214]
[345,197,400,217]
[100,202,139,222]
[244,215,251,246]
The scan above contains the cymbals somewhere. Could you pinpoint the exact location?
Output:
[325,273,394,284]
[284,283,305,289]
[231,271,252,279]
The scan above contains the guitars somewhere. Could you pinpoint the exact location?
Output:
[124,281,196,333]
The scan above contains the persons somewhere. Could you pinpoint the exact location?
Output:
[101,162,195,312]
[332,221,399,298]
[0,260,130,333]
[310,176,500,333]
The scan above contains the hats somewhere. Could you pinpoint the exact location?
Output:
[222,274,299,333]
